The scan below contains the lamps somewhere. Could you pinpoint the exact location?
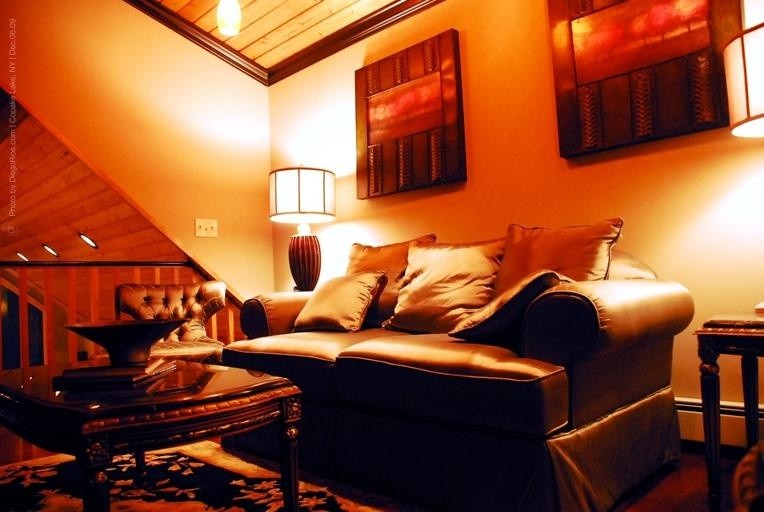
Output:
[723,23,764,316]
[216,0,241,38]
[268,166,335,291]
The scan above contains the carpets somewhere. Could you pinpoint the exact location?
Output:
[0,440,374,512]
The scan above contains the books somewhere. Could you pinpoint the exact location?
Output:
[51,358,179,406]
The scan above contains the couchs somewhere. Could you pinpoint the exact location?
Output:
[222,249,694,511]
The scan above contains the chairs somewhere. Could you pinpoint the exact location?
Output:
[90,281,224,364]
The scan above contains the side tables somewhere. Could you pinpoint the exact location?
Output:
[694,318,764,499]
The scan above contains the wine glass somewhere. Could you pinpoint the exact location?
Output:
[63,318,188,364]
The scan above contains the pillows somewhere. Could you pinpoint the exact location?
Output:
[382,236,507,336]
[492,216,623,298]
[345,234,437,324]
[446,268,577,340]
[294,270,386,333]
